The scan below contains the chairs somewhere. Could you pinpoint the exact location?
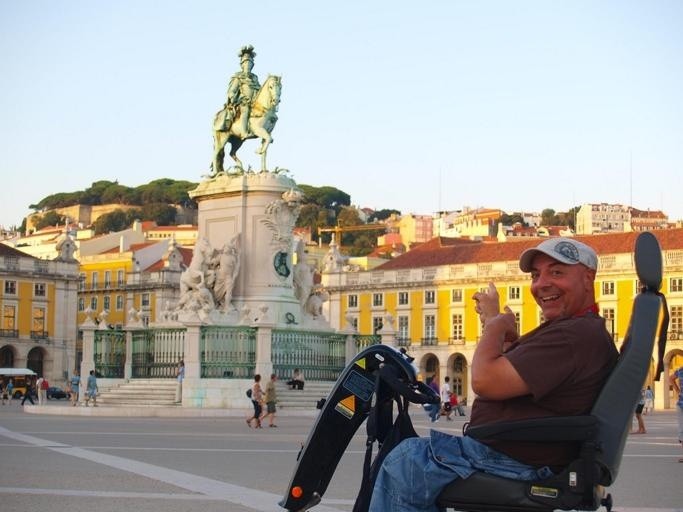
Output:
[436,230,667,512]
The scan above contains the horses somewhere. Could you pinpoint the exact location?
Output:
[212,74,282,174]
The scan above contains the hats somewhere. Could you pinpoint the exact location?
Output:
[518,237,599,273]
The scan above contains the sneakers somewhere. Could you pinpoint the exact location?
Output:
[245,419,278,429]
[431,413,466,423]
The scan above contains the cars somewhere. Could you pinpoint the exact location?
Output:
[47,387,70,399]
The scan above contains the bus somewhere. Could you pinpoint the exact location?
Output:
[0,367,38,400]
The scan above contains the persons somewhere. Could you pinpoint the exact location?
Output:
[369,236,622,511]
[258,374,280,427]
[213,245,236,313]
[20,375,49,408]
[636,389,647,433]
[227,59,262,138]
[643,385,654,415]
[292,369,304,389]
[246,375,266,429]
[0,376,14,406]
[428,374,466,422]
[172,360,184,403]
[67,370,84,406]
[669,367,683,462]
[86,370,98,408]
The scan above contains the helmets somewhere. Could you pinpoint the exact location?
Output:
[238,44,257,64]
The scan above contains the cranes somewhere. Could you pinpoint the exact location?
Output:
[318,218,390,251]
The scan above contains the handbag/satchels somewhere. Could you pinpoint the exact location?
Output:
[81,393,89,402]
[245,389,252,398]
[213,104,237,132]
